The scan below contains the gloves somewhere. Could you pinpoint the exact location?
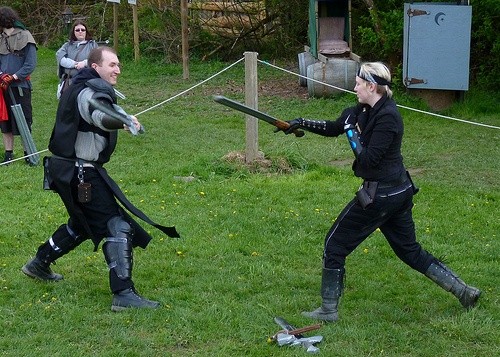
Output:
[274,118,303,135]
[343,113,357,126]
[0,73,16,90]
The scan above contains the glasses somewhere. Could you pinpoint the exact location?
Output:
[75,29,86,32]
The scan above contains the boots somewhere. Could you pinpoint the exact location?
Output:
[300,267,347,321]
[426,256,481,307]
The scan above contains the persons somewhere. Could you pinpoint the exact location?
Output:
[0,6,39,165]
[56,21,99,102]
[274,63,481,321]
[21,45,180,312]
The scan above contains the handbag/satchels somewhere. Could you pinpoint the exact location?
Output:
[60,79,70,94]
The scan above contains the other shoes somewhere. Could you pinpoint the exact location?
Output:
[0,150,13,167]
[23,151,33,167]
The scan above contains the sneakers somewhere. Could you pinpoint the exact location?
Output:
[111,286,160,313]
[23,256,64,282]
